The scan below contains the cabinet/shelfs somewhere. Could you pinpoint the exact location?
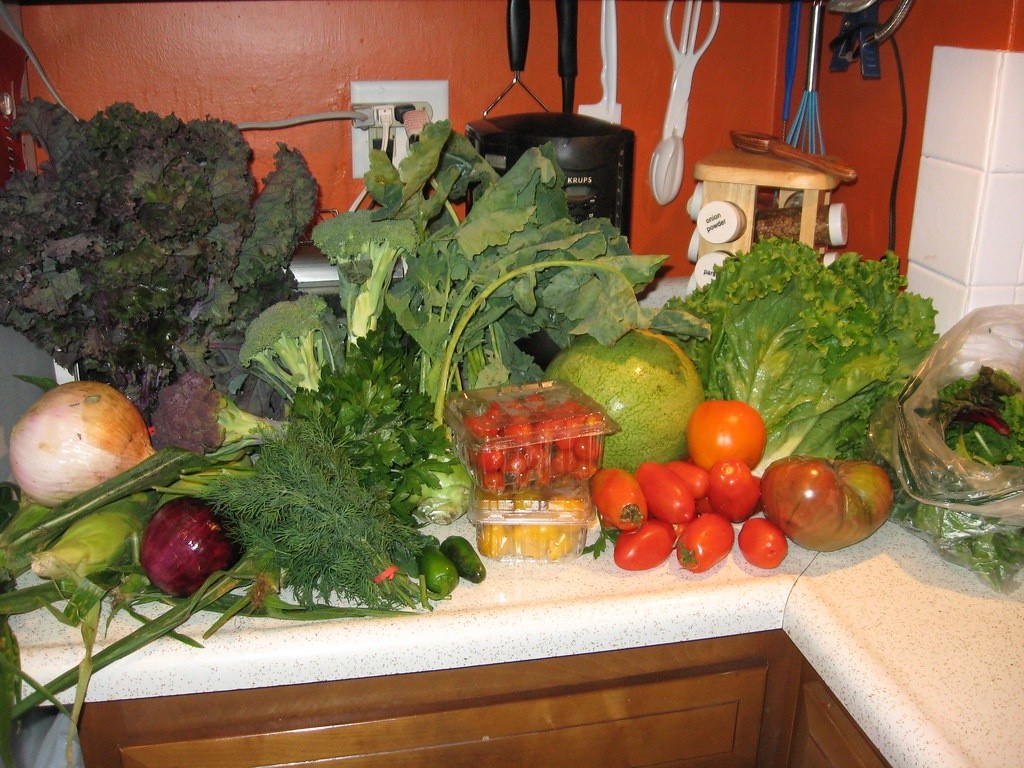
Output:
[76,628,891,768]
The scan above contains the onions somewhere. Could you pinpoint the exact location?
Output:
[141,494,244,603]
[11,380,156,509]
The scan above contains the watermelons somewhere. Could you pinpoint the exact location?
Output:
[541,325,706,477]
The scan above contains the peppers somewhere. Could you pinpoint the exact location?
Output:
[416,536,486,601]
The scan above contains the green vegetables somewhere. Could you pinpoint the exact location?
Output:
[199,330,461,612]
[868,364,1024,597]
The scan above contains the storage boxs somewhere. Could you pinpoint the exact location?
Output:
[444,377,622,498]
[467,480,597,562]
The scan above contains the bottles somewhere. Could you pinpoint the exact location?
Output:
[685,180,849,294]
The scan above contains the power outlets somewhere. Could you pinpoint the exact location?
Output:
[351,81,450,179]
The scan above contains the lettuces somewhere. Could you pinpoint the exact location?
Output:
[651,233,941,480]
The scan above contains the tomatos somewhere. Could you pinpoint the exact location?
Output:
[463,395,607,560]
[584,400,893,573]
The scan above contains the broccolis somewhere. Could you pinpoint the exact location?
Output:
[153,209,419,453]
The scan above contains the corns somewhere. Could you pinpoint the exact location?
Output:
[0,486,161,590]
